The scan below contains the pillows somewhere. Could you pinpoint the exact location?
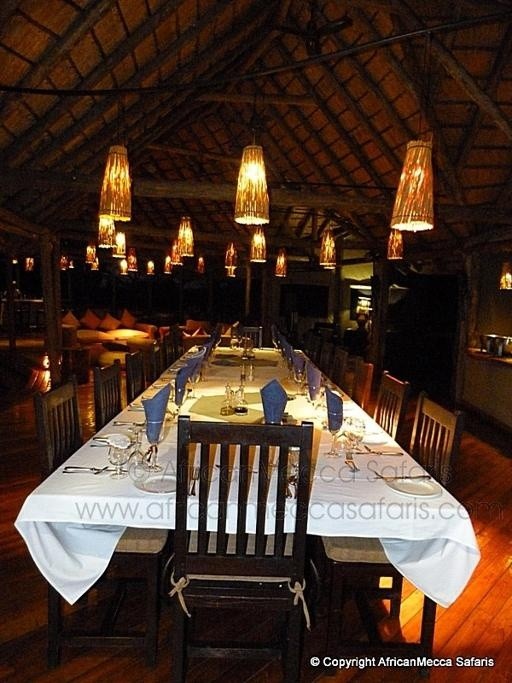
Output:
[97,312,121,330]
[121,308,137,328]
[59,311,80,329]
[80,309,101,329]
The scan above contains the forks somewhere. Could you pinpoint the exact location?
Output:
[375,471,430,478]
[63,467,108,481]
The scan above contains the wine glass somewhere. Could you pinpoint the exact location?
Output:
[113,334,368,470]
[107,444,131,481]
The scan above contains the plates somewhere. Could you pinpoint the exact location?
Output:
[136,471,177,494]
[386,476,442,498]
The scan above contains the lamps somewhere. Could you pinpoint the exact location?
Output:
[25,31,512,290]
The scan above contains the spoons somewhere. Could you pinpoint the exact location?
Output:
[364,445,400,455]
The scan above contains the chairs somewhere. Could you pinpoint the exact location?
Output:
[26,326,463,683]
